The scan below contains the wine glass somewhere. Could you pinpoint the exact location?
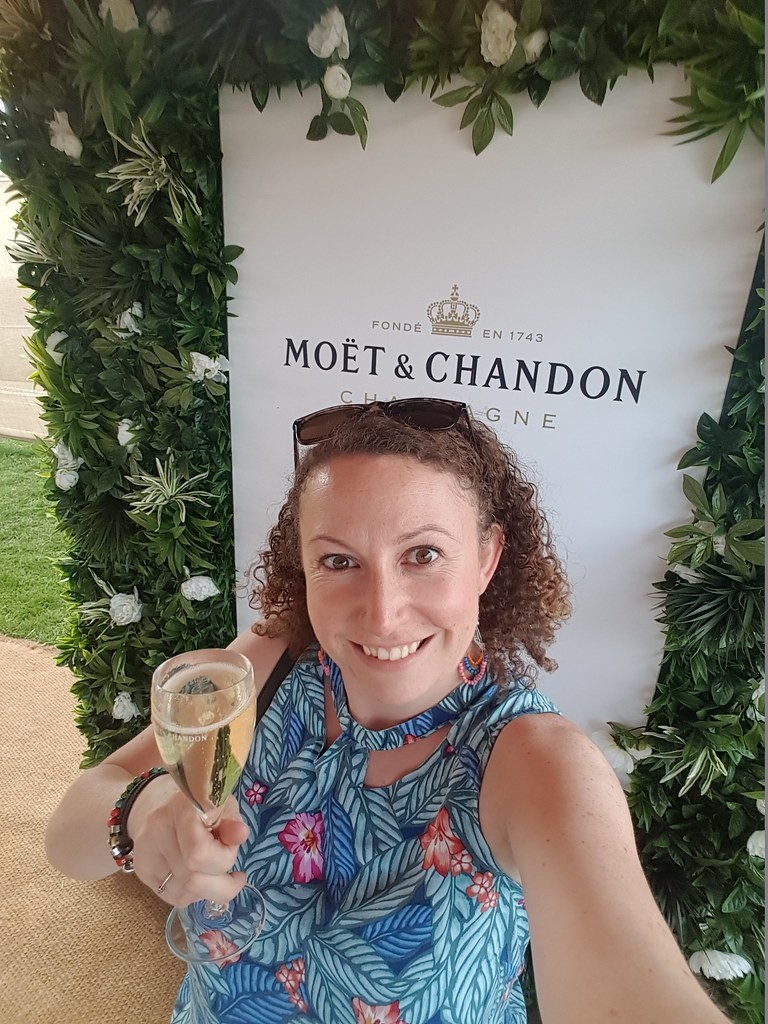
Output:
[150,648,267,965]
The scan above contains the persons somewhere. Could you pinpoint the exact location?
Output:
[45,399,733,1024]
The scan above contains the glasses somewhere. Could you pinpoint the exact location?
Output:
[293,397,479,479]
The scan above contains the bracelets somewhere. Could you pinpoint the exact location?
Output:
[106,765,170,874]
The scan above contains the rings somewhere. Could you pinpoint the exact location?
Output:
[157,873,172,894]
